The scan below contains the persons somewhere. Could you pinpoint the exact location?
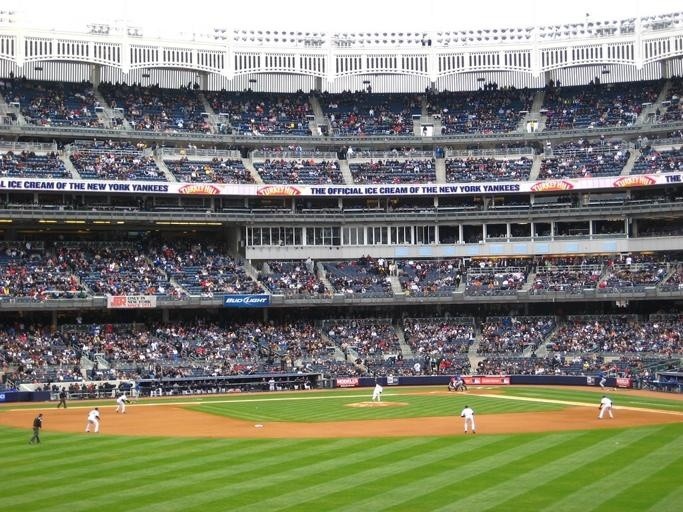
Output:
[1,80,683,401]
[460,405,476,434]
[597,396,614,419]
[114,395,131,414]
[29,414,43,443]
[85,408,101,433]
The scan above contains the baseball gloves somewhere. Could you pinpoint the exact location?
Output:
[599,406,602,409]
